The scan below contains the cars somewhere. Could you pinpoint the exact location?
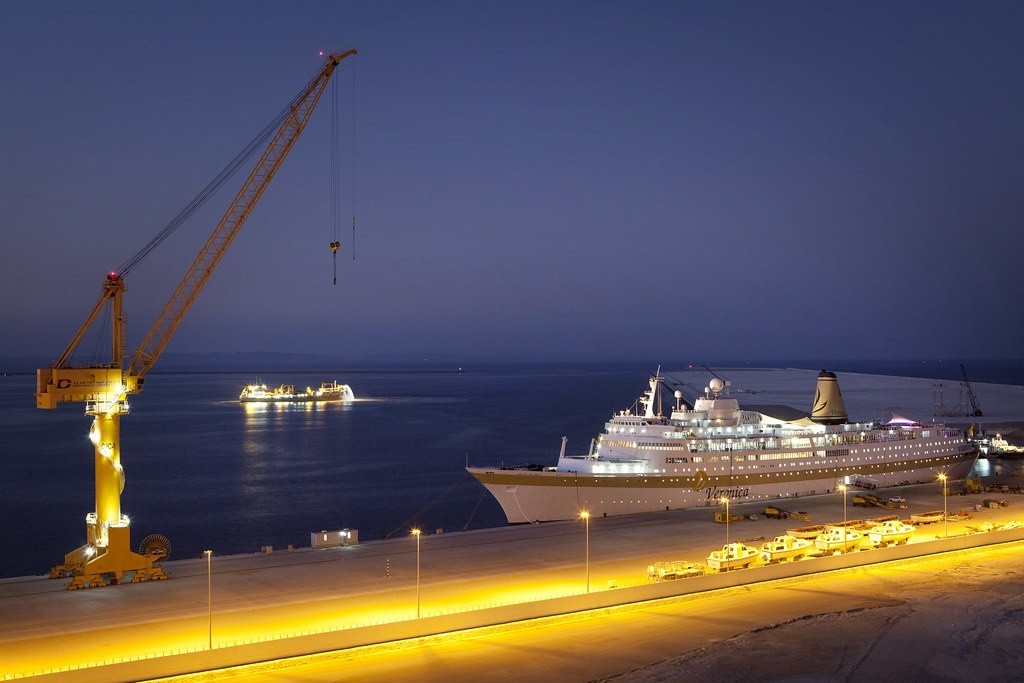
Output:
[889,496,906,503]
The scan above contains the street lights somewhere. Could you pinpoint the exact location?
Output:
[578,508,590,594]
[936,471,949,538]
[719,495,731,572]
[409,525,421,619]
[203,549,213,650]
[836,484,847,556]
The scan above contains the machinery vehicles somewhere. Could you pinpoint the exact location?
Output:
[851,493,897,511]
[759,505,810,522]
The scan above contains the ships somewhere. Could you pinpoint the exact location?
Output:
[463,357,982,527]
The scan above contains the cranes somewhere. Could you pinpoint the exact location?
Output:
[35,47,361,594]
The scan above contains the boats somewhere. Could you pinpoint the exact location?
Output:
[827,520,863,526]
[868,520,917,542]
[814,527,862,550]
[865,514,899,523]
[708,541,759,570]
[909,509,945,523]
[237,378,354,402]
[762,534,813,559]
[784,525,824,538]
